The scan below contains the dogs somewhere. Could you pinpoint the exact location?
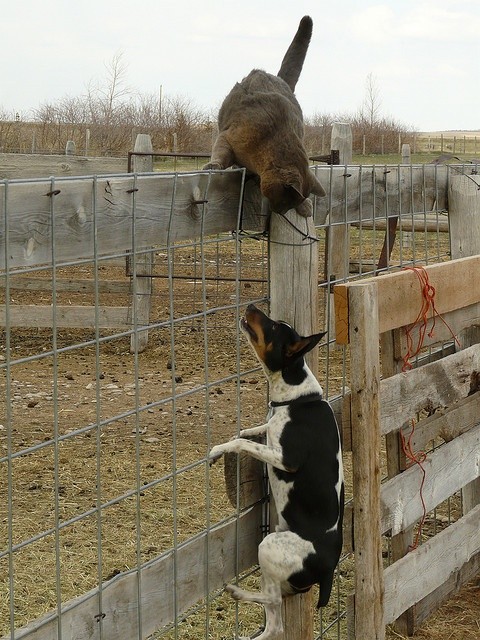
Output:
[207,302,344,639]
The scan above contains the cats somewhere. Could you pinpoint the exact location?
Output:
[202,13,328,217]
